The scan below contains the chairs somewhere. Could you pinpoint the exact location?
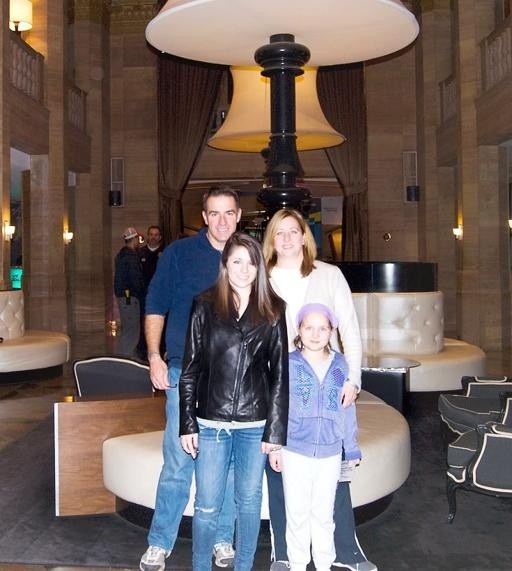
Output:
[73,355,156,399]
[444,392,512,525]
[437,376,512,461]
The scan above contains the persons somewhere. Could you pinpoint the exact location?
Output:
[178,232,285,571]
[113,227,144,357]
[269,305,362,571]
[138,184,244,571]
[261,207,379,571]
[138,225,166,360]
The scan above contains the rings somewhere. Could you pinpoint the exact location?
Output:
[272,449,277,451]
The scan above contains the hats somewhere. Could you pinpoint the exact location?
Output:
[122,227,145,241]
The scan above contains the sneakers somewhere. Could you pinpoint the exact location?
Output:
[213,542,235,569]
[332,561,378,571]
[270,561,290,571]
[139,545,171,571]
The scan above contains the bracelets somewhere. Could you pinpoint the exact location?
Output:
[346,377,359,390]
[147,352,161,362]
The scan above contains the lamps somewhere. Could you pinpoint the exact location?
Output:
[108,190,121,207]
[9,0,32,35]
[206,66,347,153]
[63,233,74,246]
[453,228,463,240]
[4,221,15,240]
[144,0,420,226]
[407,186,420,202]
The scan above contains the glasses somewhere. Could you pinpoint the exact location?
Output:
[152,356,181,387]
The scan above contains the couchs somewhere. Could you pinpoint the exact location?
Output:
[350,290,487,394]
[0,289,72,385]
[102,367,413,552]
